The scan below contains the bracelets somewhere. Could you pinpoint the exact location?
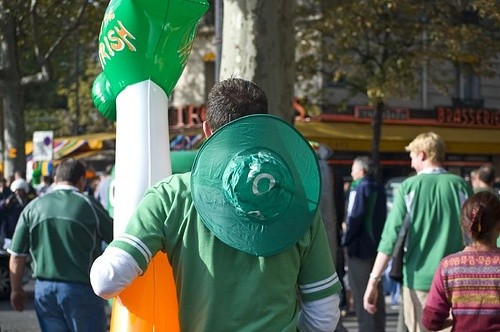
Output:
[370,273,383,283]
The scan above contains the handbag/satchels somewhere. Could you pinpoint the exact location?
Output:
[384,195,412,281]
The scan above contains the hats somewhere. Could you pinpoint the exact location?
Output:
[190,115,322,257]
[10,179,29,193]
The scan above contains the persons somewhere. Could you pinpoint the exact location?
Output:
[468,163,500,198]
[422,190,500,332]
[342,156,388,331]
[88,78,346,331]
[0,159,116,331]
[361,131,473,332]
